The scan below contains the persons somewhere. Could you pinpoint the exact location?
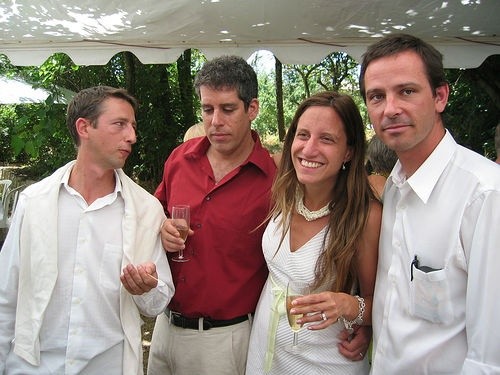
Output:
[364,132,400,205]
[245,91,382,375]
[359,33,500,375]
[147,55,372,375]
[0,86,175,375]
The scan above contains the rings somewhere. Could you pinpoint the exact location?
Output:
[359,353,365,358]
[319,312,328,321]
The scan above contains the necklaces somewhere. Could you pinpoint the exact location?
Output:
[295,194,338,222]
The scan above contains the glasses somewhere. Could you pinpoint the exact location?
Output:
[365,160,388,179]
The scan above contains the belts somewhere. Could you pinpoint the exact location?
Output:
[162,308,255,330]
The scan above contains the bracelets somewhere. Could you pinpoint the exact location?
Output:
[338,295,365,333]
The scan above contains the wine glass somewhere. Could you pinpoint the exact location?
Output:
[171,205,191,263]
[282,281,311,354]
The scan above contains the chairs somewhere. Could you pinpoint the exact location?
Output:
[0,180,26,240]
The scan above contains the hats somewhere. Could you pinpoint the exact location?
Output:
[183,122,206,143]
